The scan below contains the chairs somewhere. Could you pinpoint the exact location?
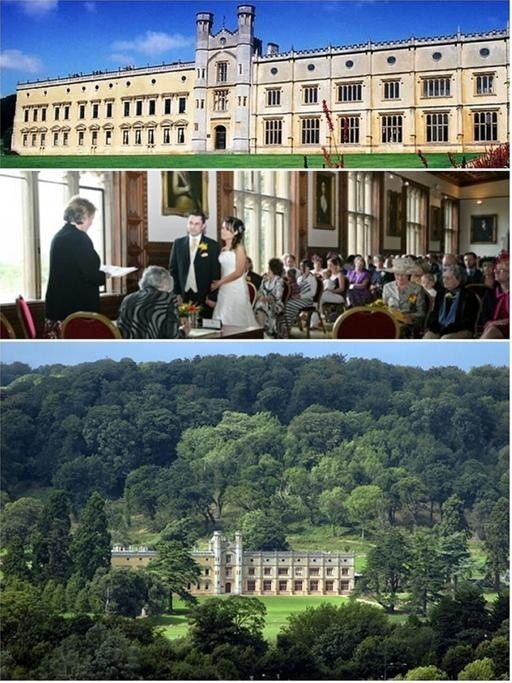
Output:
[0,275,495,339]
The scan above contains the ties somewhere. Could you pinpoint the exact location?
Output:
[190,239,196,249]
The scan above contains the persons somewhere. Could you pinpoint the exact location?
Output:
[44,197,111,320]
[118,209,510,341]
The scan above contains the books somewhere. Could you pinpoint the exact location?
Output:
[96,262,136,279]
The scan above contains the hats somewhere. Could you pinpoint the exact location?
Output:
[385,258,422,274]
[479,254,497,268]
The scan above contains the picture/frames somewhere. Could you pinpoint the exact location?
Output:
[467,211,498,245]
[160,170,211,222]
[309,171,339,230]
[384,186,446,242]
